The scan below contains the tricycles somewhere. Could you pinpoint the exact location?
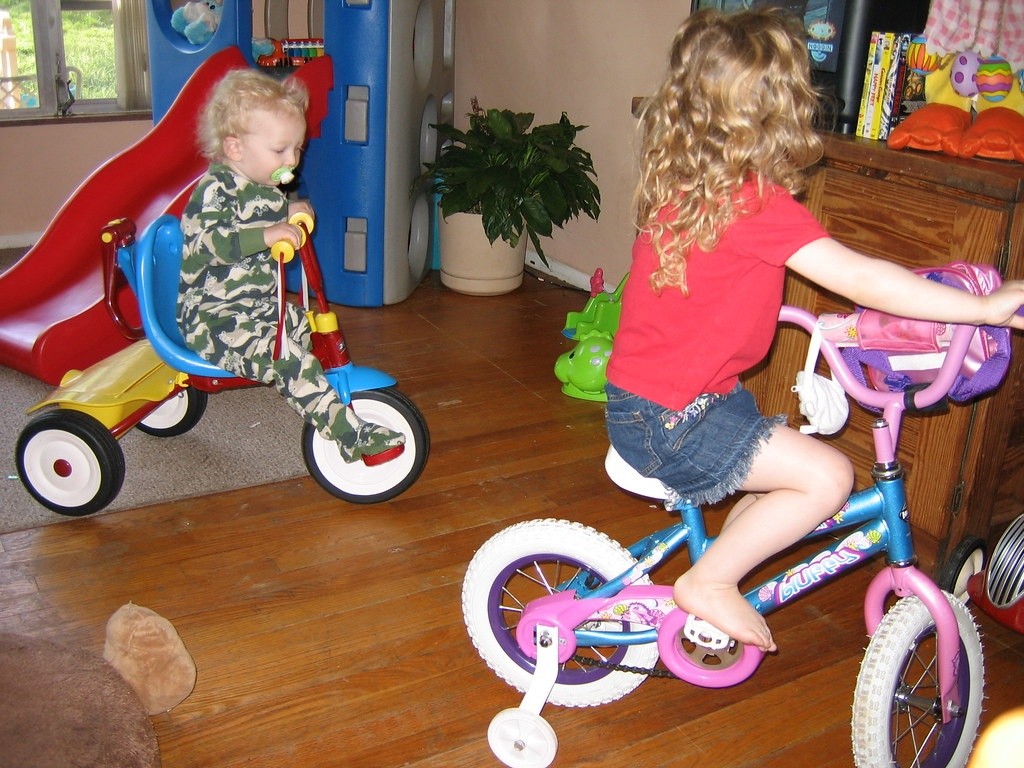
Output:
[14,207,435,516]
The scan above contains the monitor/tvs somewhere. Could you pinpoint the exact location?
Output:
[692,0,931,136]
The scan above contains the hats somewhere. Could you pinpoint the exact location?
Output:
[103,601,196,716]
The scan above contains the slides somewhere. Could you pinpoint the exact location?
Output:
[0,47,333,386]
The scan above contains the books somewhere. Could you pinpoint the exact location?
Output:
[855,31,925,141]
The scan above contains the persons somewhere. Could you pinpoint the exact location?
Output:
[604,9,1024,654]
[176,69,406,464]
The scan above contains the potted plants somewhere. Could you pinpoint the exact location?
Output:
[408,94,601,297]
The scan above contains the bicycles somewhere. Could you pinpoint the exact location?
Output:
[461,303,1023,767]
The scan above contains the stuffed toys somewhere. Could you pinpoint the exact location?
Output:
[171,0,224,44]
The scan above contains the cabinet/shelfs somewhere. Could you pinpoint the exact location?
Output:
[630,96,1023,586]
